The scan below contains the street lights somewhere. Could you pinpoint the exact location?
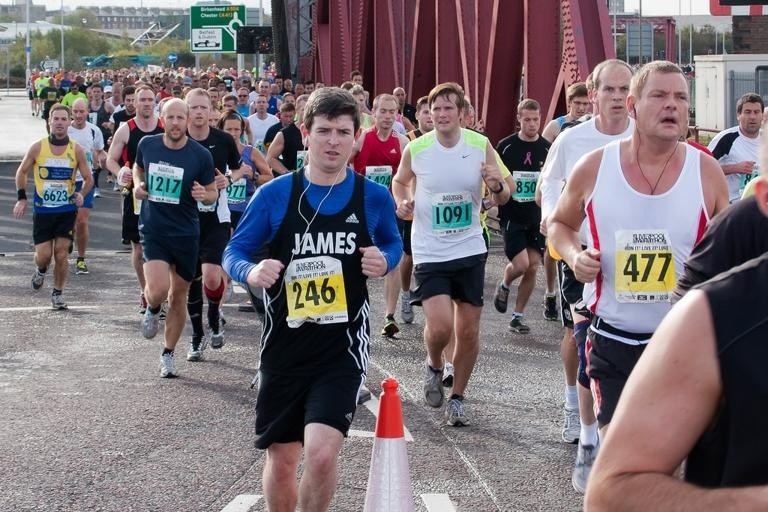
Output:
[12,18,18,42]
[77,15,88,26]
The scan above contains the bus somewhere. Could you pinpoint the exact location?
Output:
[78,54,163,67]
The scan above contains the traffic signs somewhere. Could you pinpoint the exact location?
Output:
[40,61,58,71]
[189,4,246,53]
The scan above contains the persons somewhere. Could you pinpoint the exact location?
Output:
[494,98,554,336]
[15,104,95,310]
[669,187,766,303]
[391,83,510,427]
[219,86,400,512]
[708,91,767,221]
[583,177,766,512]
[26,60,517,388]
[541,81,596,322]
[545,58,731,497]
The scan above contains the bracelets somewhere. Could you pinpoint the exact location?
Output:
[17,188,27,200]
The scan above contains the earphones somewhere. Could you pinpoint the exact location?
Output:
[353,138,356,148]
[304,135,309,147]
[632,104,636,119]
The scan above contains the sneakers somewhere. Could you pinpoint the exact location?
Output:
[493,281,558,333]
[571,432,600,495]
[93,188,102,198]
[30,265,49,289]
[49,289,69,308]
[105,175,115,184]
[381,290,471,426]
[75,260,90,275]
[561,400,580,444]
[139,290,233,377]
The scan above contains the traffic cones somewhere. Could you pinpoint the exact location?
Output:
[361,378,417,512]
